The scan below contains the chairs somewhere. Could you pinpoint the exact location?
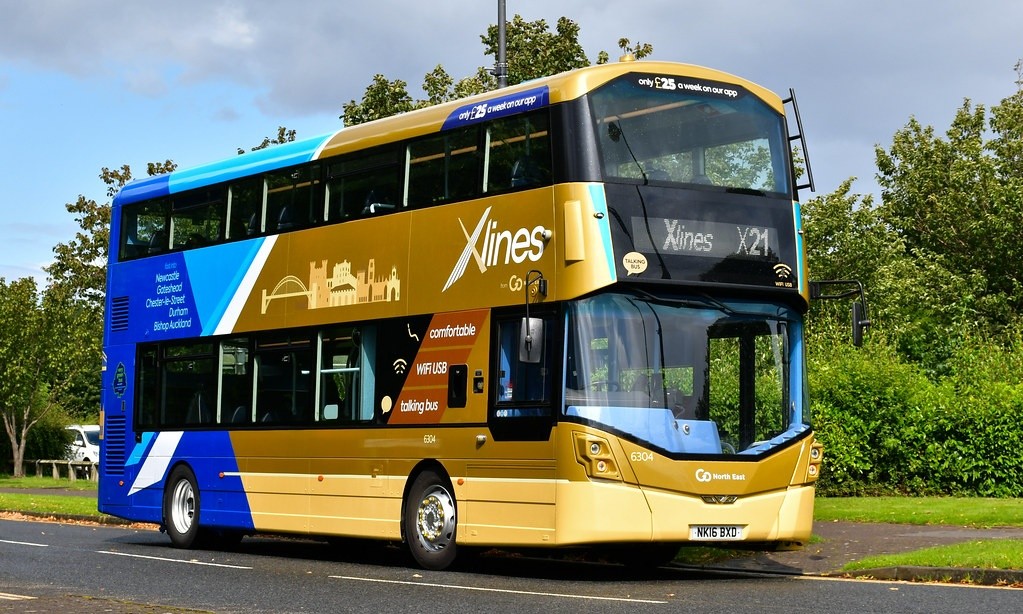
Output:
[146,201,308,257]
[259,402,297,427]
[228,406,250,422]
[364,184,398,214]
[510,155,549,189]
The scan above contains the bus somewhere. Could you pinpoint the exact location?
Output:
[96,51,870,568]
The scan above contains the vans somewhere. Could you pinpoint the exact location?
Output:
[63,425,101,480]
